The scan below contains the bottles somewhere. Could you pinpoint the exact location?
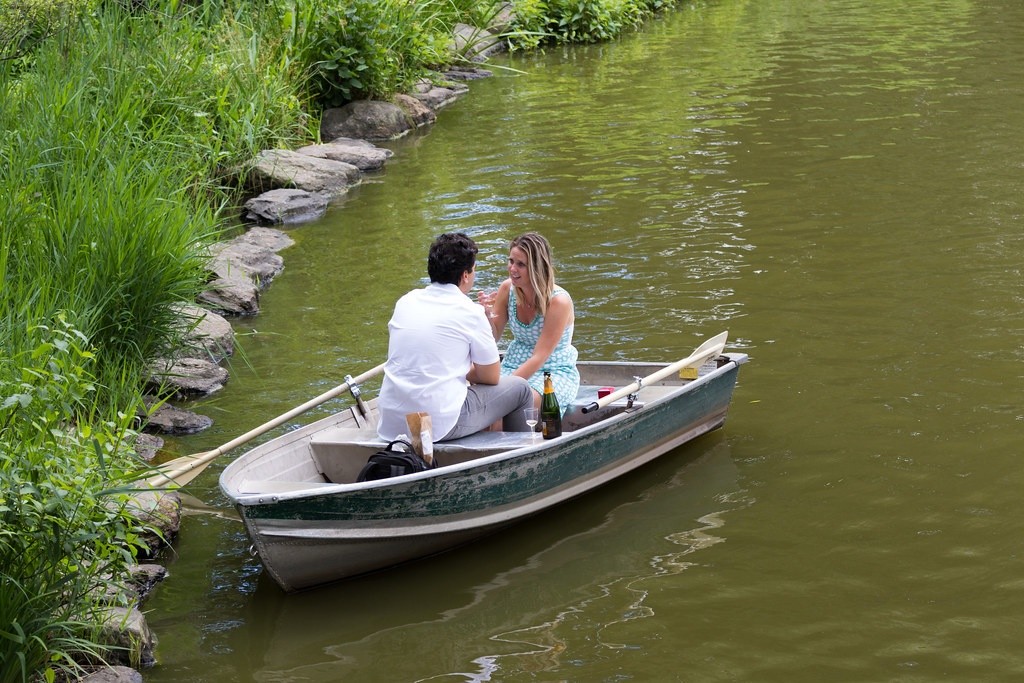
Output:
[541,371,562,439]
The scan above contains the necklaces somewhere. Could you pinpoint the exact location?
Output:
[526,303,531,308]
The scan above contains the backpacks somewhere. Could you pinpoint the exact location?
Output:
[355,441,439,482]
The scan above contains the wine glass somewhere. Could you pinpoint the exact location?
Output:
[523,407,539,439]
[483,287,500,318]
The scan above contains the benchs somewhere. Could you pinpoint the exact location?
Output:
[309,427,575,484]
[558,384,681,432]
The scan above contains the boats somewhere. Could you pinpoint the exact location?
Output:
[218,344,749,592]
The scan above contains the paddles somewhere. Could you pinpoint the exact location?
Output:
[578,328,731,415]
[136,359,388,494]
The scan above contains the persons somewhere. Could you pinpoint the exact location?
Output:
[477,231,581,431]
[377,233,534,443]
